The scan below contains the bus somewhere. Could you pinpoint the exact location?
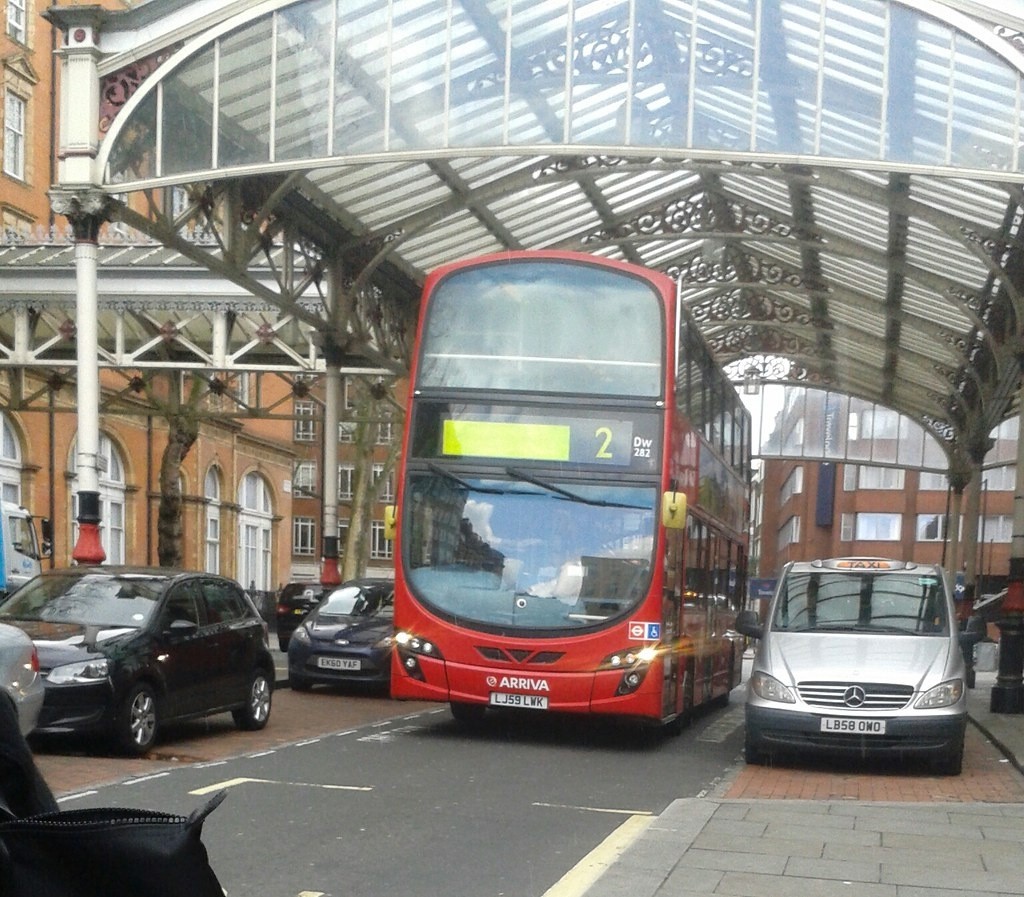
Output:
[383,246,756,737]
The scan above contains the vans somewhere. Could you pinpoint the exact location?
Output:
[730,551,984,775]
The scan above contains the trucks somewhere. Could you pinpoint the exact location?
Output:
[1,501,56,614]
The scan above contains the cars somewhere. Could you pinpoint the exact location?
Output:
[275,582,335,652]
[283,577,400,695]
[0,622,45,741]
[0,561,276,759]
